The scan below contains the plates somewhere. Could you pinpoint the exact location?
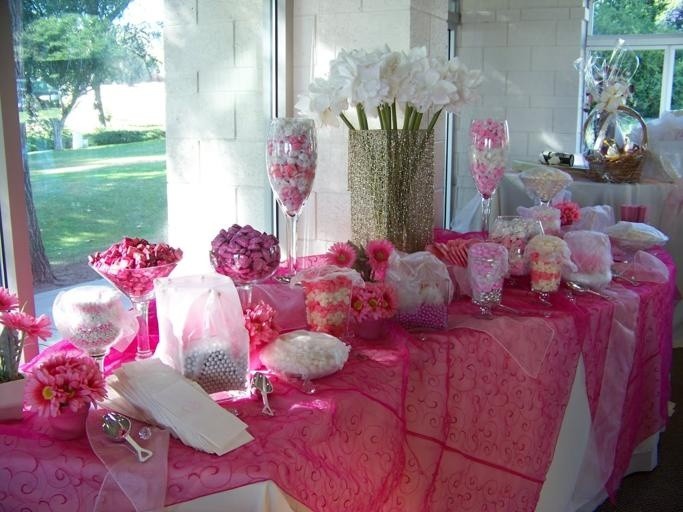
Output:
[256,329,349,382]
[607,235,669,254]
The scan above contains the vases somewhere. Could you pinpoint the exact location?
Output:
[0,371,27,421]
[350,316,392,342]
[45,405,88,442]
[347,129,435,264]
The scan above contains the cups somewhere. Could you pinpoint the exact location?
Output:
[488,215,547,279]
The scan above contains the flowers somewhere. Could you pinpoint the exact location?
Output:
[242,303,279,349]
[329,238,396,320]
[294,35,485,220]
[0,286,51,382]
[555,203,579,226]
[585,84,633,118]
[22,352,109,418]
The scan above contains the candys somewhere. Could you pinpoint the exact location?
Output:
[525,169,564,201]
[469,119,506,197]
[183,341,248,395]
[52,284,123,351]
[530,239,563,293]
[268,334,349,379]
[87,236,183,297]
[469,244,506,306]
[397,302,448,330]
[494,217,532,276]
[303,274,353,337]
[209,224,281,283]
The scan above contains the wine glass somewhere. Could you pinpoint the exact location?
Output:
[517,173,572,208]
[467,242,510,321]
[468,119,509,232]
[205,244,281,321]
[86,260,178,363]
[527,242,563,308]
[264,118,318,286]
[56,284,123,383]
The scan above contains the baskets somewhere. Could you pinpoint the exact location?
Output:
[582,105,648,182]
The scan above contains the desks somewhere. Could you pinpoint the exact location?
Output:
[503,163,683,347]
[0,228,683,512]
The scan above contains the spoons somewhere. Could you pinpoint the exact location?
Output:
[98,410,153,463]
[564,278,612,303]
[248,370,274,417]
